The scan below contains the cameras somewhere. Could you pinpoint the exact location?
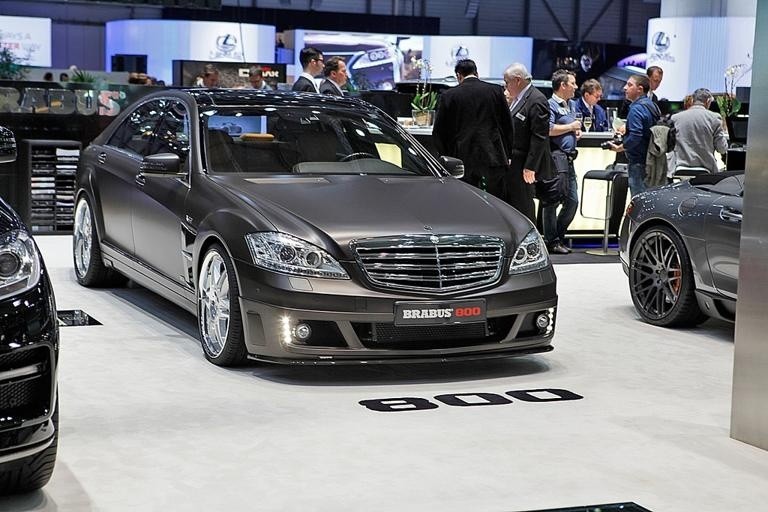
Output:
[600,135,623,149]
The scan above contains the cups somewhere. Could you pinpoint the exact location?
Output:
[574,113,582,123]
[606,106,618,131]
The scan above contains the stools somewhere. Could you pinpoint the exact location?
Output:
[577,163,710,260]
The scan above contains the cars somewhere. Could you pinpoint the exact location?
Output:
[618,169,744,327]
[0,125,60,497]
[305,32,409,90]
[73,87,559,369]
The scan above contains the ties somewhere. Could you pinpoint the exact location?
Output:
[509,99,518,111]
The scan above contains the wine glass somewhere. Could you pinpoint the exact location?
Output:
[583,116,592,134]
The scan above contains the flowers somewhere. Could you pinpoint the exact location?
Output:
[407,57,436,111]
[715,65,742,120]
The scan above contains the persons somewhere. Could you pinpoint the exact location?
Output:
[574,78,612,132]
[243,66,272,92]
[542,69,583,254]
[619,65,664,120]
[501,63,559,230]
[682,95,731,174]
[319,55,350,97]
[290,45,325,95]
[59,73,69,81]
[190,63,228,89]
[606,74,665,199]
[44,72,53,82]
[665,87,730,180]
[429,59,514,206]
[502,83,515,108]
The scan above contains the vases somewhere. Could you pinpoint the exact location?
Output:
[411,108,435,128]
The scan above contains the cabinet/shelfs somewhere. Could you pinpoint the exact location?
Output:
[19,139,83,236]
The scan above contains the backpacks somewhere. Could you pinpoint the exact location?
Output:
[643,102,676,153]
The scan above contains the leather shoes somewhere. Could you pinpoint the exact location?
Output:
[552,246,568,254]
[563,245,572,253]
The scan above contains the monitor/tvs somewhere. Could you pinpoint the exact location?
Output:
[180,60,288,90]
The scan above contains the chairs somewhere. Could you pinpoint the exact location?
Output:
[207,128,339,173]
